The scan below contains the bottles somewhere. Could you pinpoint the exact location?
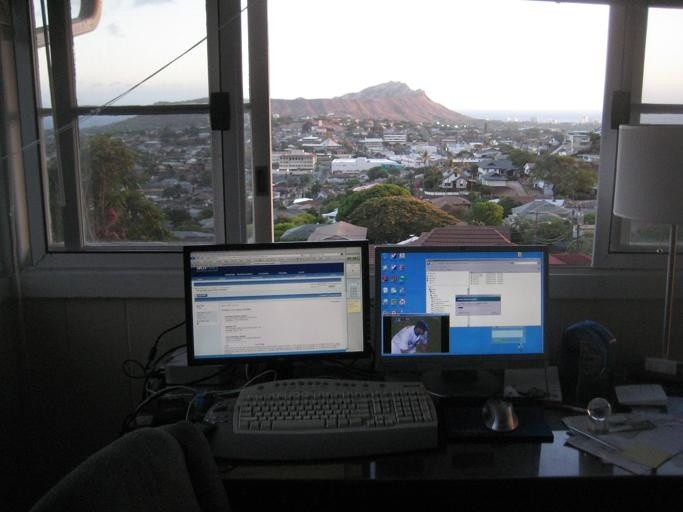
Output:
[417,321,429,332]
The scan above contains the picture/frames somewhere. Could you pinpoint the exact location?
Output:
[150,371,681,511]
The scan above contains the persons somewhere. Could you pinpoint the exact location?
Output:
[390,320,429,353]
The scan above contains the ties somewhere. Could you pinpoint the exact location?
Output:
[374,245,550,399]
[182,241,369,372]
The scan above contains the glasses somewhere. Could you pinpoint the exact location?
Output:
[612,122,683,375]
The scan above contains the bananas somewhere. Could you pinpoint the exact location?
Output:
[232,378,440,435]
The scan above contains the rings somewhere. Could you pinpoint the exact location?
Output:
[567,425,618,450]
[561,405,589,413]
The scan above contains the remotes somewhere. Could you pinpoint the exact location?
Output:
[481,394,520,432]
